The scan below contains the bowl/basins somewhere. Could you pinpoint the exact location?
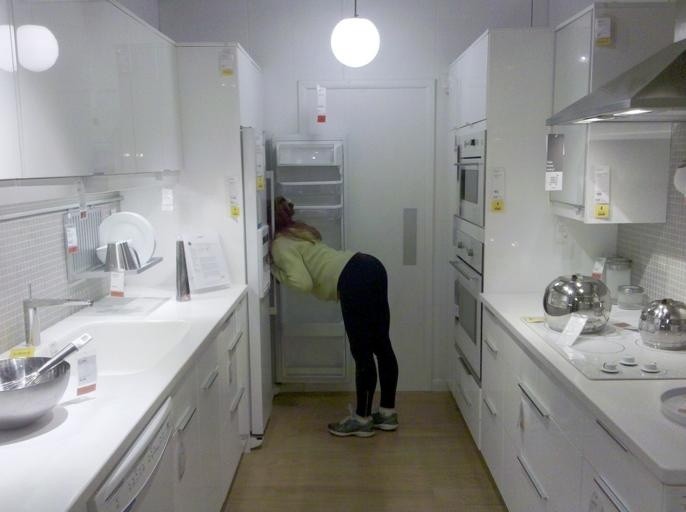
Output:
[0,357,71,432]
[96,245,108,265]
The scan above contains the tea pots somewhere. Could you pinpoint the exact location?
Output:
[104,241,141,270]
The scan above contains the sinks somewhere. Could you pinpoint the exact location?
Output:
[34,319,189,376]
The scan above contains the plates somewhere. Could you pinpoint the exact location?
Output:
[98,211,156,267]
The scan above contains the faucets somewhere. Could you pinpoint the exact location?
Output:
[21,282,94,349]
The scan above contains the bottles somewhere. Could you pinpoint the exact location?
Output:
[604,257,632,304]
[618,285,644,310]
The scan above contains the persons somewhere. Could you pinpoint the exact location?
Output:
[267,196,399,436]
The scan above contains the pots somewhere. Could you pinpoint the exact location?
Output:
[638,299,686,350]
[543,274,611,335]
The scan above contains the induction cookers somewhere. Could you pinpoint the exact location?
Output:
[521,314,686,381]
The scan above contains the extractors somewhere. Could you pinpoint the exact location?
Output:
[545,38,686,128]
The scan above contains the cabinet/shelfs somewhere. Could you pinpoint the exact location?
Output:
[575,412,686,512]
[172,314,252,512]
[497,347,573,512]
[546,1,671,226]
[330,0,382,67]
[1,0,183,179]
[475,310,519,477]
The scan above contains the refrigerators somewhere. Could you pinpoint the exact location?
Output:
[243,127,354,436]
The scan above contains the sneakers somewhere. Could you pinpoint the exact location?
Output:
[371,411,400,430]
[328,415,375,437]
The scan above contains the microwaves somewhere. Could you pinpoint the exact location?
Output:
[453,130,486,228]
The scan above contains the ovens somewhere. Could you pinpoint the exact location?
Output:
[452,215,484,390]
